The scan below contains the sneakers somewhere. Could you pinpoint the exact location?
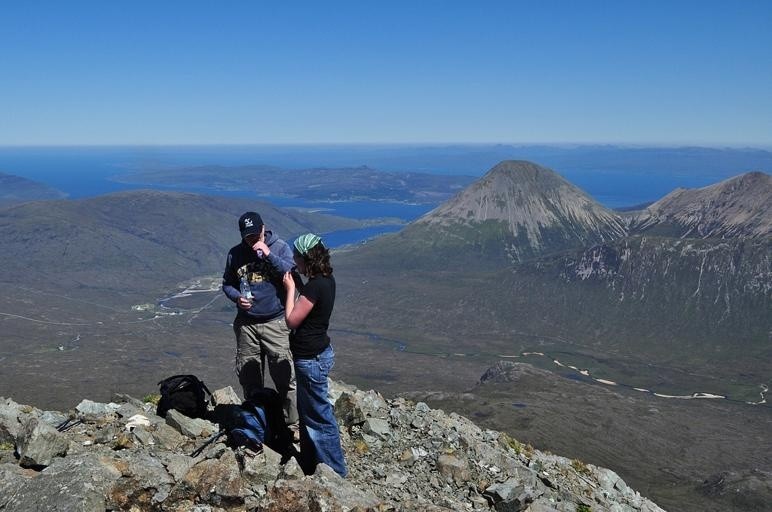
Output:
[288,423,300,442]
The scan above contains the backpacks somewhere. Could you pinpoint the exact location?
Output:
[229,387,295,456]
[157,375,209,419]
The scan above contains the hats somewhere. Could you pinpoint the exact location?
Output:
[239,212,263,239]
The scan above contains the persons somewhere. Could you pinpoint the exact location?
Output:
[222,212,299,444]
[282,233,347,478]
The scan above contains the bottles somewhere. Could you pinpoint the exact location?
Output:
[239,277,253,307]
